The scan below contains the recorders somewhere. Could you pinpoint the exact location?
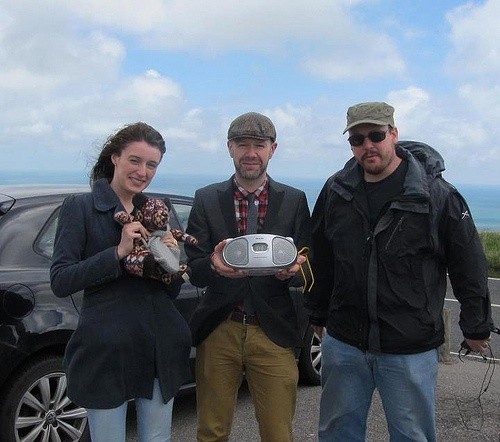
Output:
[219,233,298,277]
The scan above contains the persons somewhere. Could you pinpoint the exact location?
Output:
[49,121,192,442]
[311,102,498,442]
[183,112,313,442]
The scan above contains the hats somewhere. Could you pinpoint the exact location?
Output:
[227,112,276,142]
[343,102,394,135]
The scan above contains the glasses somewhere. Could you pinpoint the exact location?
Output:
[348,126,391,146]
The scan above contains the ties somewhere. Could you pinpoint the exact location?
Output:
[244,194,256,315]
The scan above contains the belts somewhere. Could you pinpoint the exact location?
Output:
[230,313,260,326]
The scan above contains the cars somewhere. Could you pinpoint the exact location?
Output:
[0,185,326,442]
